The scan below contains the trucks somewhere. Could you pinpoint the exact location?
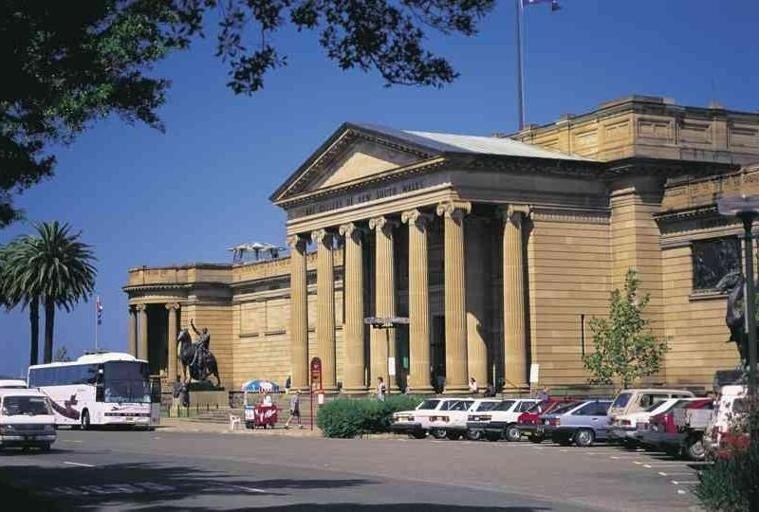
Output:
[0,379,59,455]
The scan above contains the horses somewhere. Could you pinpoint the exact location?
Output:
[177,328,221,386]
[716,266,759,370]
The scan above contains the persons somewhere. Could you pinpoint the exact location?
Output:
[284,375,291,395]
[484,380,498,397]
[376,376,388,401]
[536,385,550,400]
[190,317,210,367]
[469,377,479,394]
[283,389,304,429]
[261,391,273,404]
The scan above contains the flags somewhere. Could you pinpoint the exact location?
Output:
[97,295,103,325]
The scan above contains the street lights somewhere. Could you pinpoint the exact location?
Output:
[363,313,412,396]
[715,187,758,445]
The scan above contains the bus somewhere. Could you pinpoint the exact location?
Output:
[145,374,163,432]
[24,352,155,432]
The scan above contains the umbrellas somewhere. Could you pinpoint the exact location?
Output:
[241,379,280,394]
